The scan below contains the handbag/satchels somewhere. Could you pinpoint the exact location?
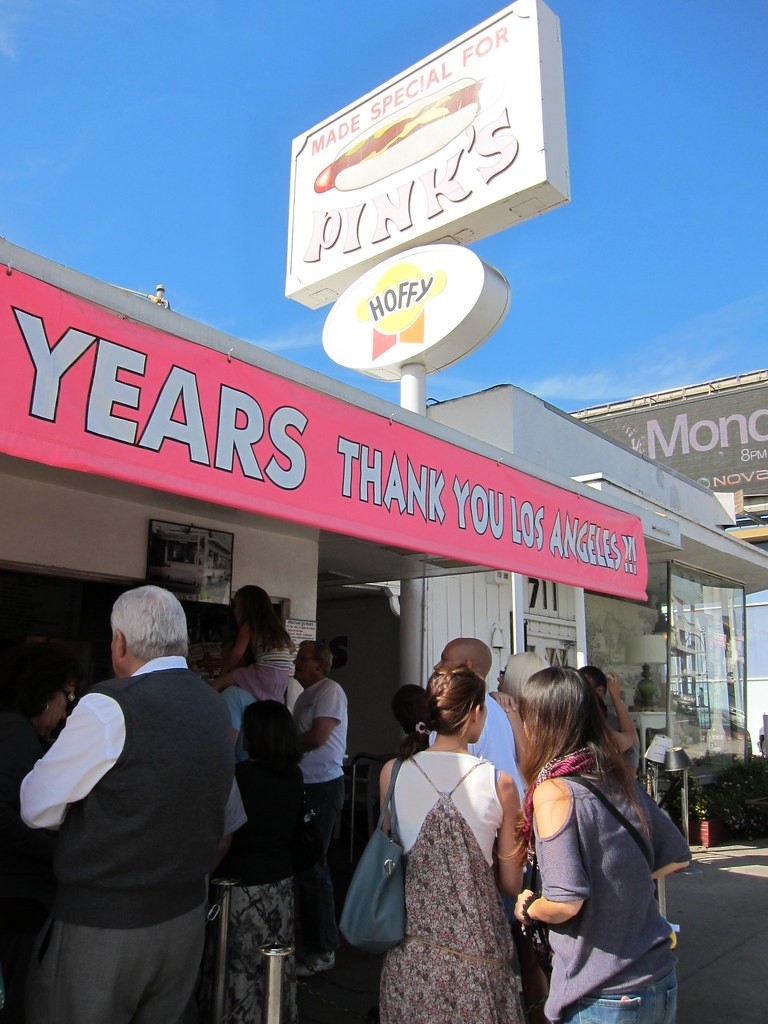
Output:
[339,756,408,952]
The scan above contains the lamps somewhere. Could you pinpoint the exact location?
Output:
[663,748,694,772]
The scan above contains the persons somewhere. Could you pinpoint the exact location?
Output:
[377,638,690,1024]
[0,584,349,1024]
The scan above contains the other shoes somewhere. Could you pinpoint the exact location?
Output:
[294,951,335,977]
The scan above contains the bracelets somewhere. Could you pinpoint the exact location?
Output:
[520,891,544,935]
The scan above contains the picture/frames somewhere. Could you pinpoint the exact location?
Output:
[145,518,233,607]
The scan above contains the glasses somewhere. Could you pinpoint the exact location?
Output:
[297,655,322,663]
[61,685,77,706]
